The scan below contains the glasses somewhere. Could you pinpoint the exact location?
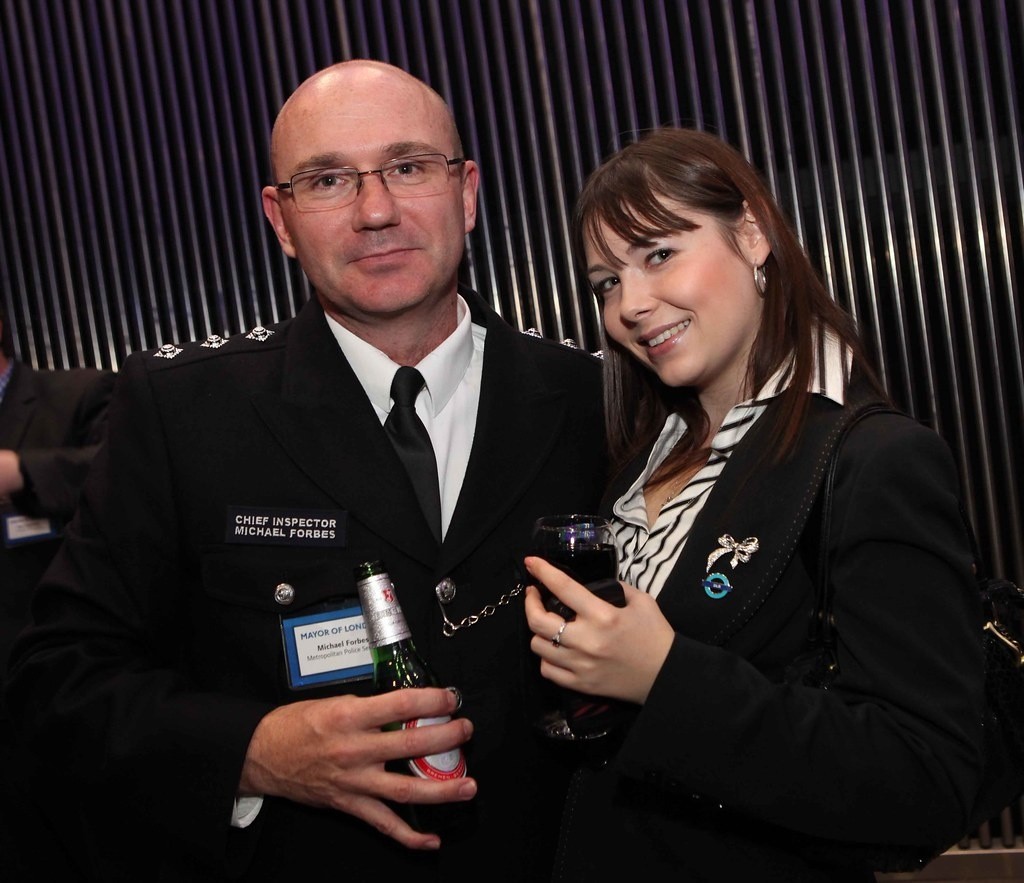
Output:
[275,154,465,213]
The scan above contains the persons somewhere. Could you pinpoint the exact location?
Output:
[0,318,122,637]
[93,58,635,883]
[522,123,989,883]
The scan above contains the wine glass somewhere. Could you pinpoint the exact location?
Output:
[535,509,619,739]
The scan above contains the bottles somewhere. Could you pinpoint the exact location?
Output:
[352,554,500,883]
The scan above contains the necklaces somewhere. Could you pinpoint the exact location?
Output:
[660,462,708,506]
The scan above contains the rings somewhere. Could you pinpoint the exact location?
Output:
[551,623,567,645]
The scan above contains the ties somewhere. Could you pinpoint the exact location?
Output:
[381,365,442,552]
